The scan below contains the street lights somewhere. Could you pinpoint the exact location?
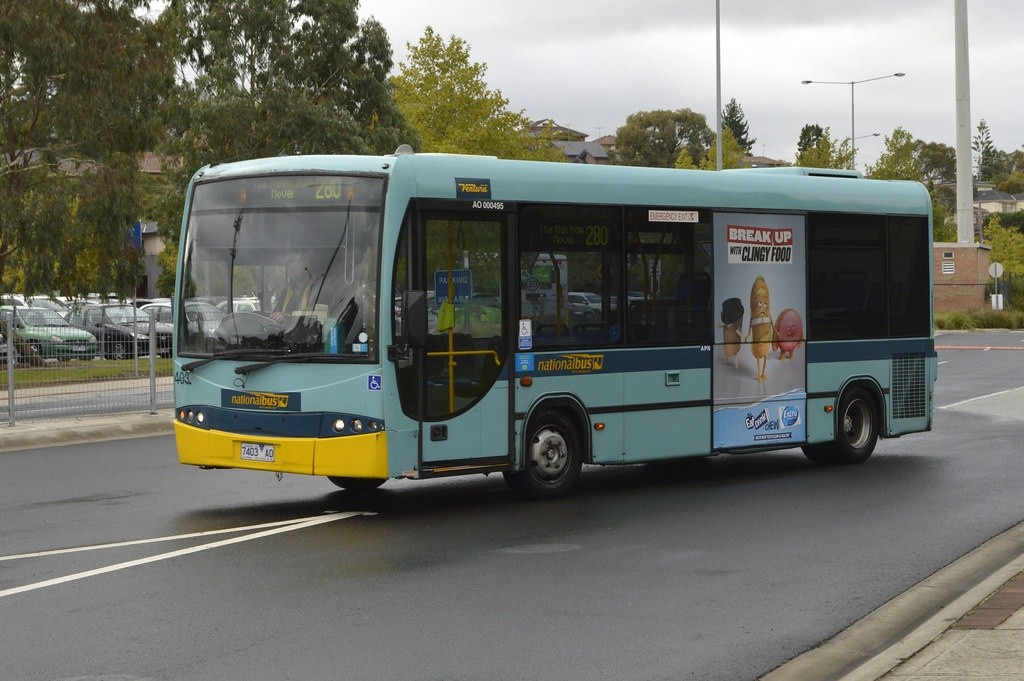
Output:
[800,72,908,169]
[846,132,880,169]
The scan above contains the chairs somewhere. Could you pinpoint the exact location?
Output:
[428,269,712,382]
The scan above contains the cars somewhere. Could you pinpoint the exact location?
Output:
[0,290,503,341]
[566,287,654,320]
[64,301,173,359]
[0,305,100,361]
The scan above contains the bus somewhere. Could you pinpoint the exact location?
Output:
[521,253,571,328]
[169,143,937,503]
